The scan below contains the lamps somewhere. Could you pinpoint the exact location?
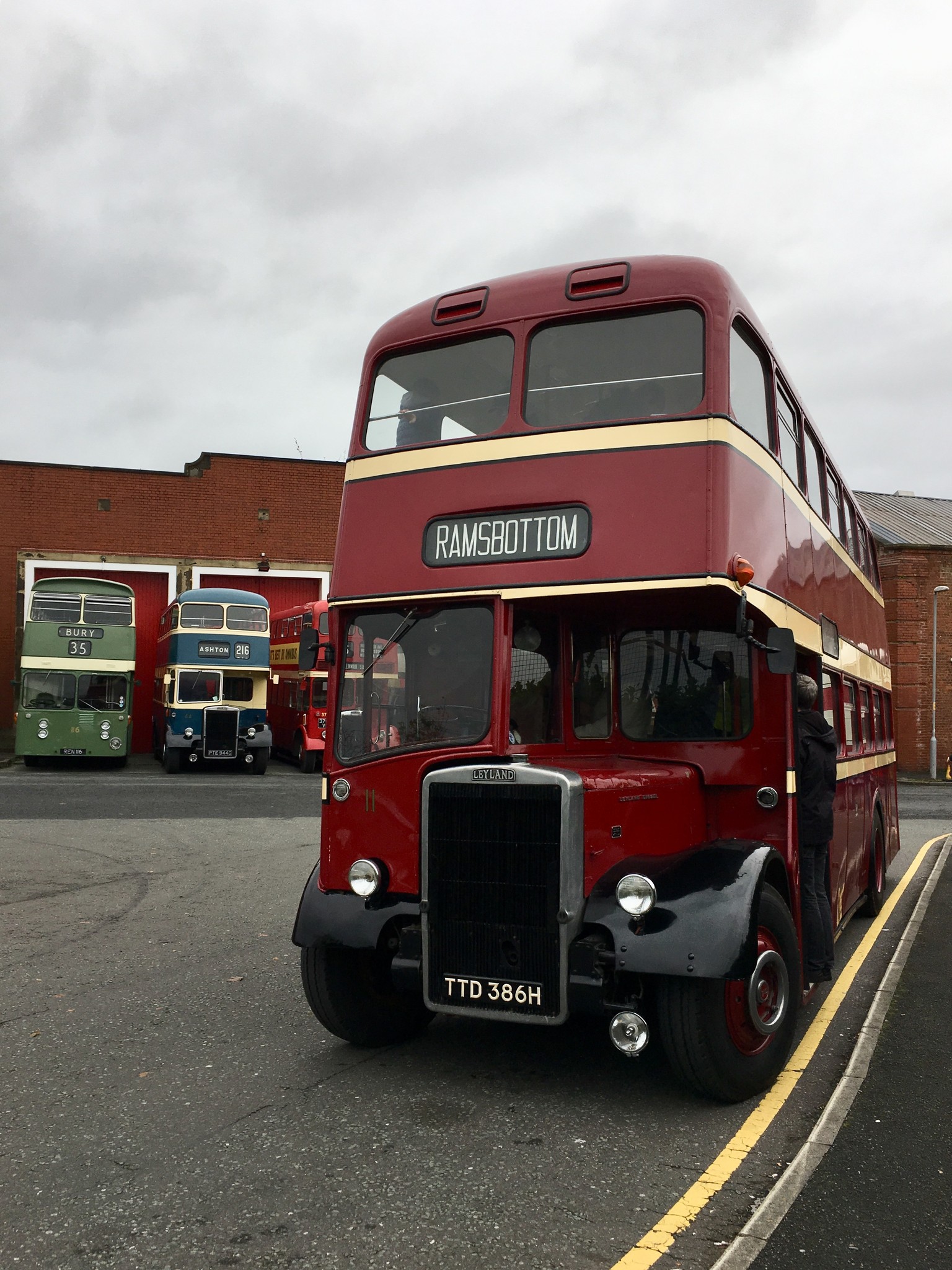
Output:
[259,562,269,572]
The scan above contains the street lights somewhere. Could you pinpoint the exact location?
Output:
[928,585,951,778]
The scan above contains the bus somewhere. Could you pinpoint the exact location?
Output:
[152,587,280,777]
[292,256,903,1105]
[266,599,402,774]
[10,576,142,771]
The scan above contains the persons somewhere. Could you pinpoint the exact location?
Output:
[788,671,838,982]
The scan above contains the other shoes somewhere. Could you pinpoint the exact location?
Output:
[803,967,831,984]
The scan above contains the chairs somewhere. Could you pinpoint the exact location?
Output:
[508,689,548,743]
[35,692,53,709]
[190,684,207,699]
